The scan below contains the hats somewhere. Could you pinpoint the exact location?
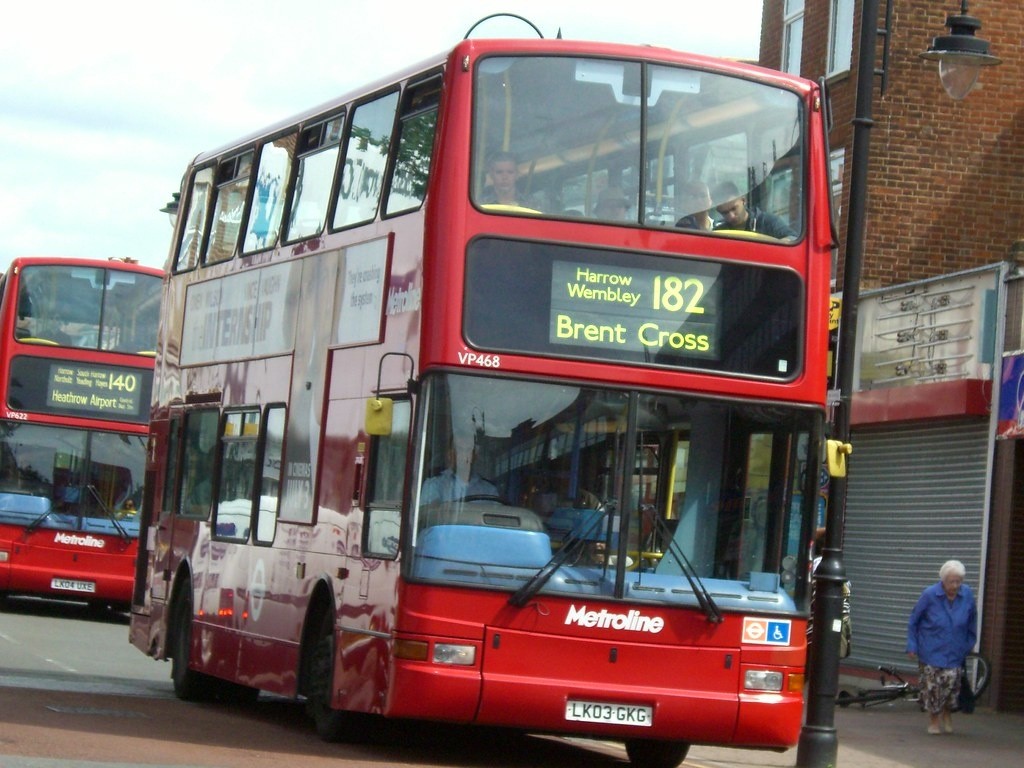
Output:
[593,188,631,215]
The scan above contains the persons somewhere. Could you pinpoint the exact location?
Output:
[712,183,797,240]
[907,559,979,734]
[675,180,721,230]
[477,152,541,210]
[593,185,631,221]
[538,458,633,567]
[419,432,500,509]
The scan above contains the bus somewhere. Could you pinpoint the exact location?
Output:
[126,35,854,768]
[0,257,164,606]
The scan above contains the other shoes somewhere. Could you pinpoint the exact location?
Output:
[928,728,942,734]
[944,726,953,733]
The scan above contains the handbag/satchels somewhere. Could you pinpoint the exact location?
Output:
[952,663,975,713]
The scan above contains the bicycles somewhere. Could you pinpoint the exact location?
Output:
[835,650,992,712]
